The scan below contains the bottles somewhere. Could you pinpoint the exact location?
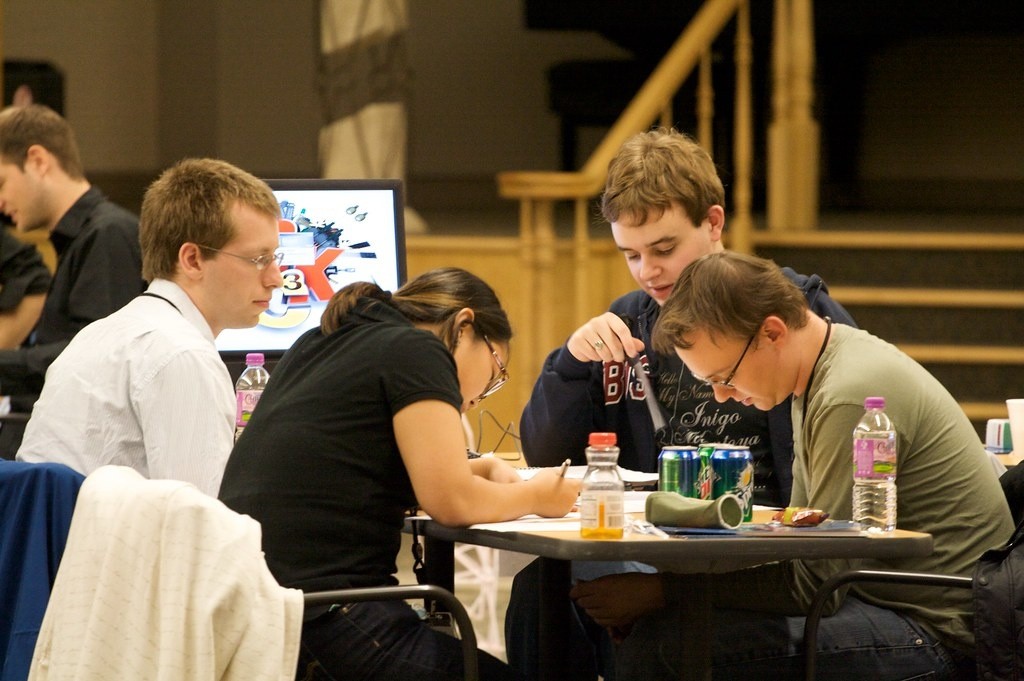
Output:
[234,353,270,443]
[579,432,625,541]
[852,396,898,533]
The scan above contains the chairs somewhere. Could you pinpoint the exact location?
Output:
[805,460,1024,681]
[1,461,479,681]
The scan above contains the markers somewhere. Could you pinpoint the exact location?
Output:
[629,353,668,432]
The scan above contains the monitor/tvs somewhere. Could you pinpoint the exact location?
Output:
[214,179,407,360]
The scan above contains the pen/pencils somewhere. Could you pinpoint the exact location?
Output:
[559,458,571,477]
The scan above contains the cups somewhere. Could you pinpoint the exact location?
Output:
[1005,399,1024,456]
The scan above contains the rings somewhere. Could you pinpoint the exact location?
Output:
[593,340,604,348]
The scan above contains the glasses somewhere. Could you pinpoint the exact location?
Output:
[476,325,510,402]
[198,244,284,271]
[690,315,768,391]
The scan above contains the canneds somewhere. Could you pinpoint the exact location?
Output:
[657,442,754,523]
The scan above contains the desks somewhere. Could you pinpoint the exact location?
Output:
[402,511,936,681]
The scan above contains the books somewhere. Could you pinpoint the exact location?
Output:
[513,464,659,486]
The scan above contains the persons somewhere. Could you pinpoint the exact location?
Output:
[216,265,582,681]
[505,126,864,681]
[649,252,1018,681]
[15,157,285,500]
[0,104,149,462]
